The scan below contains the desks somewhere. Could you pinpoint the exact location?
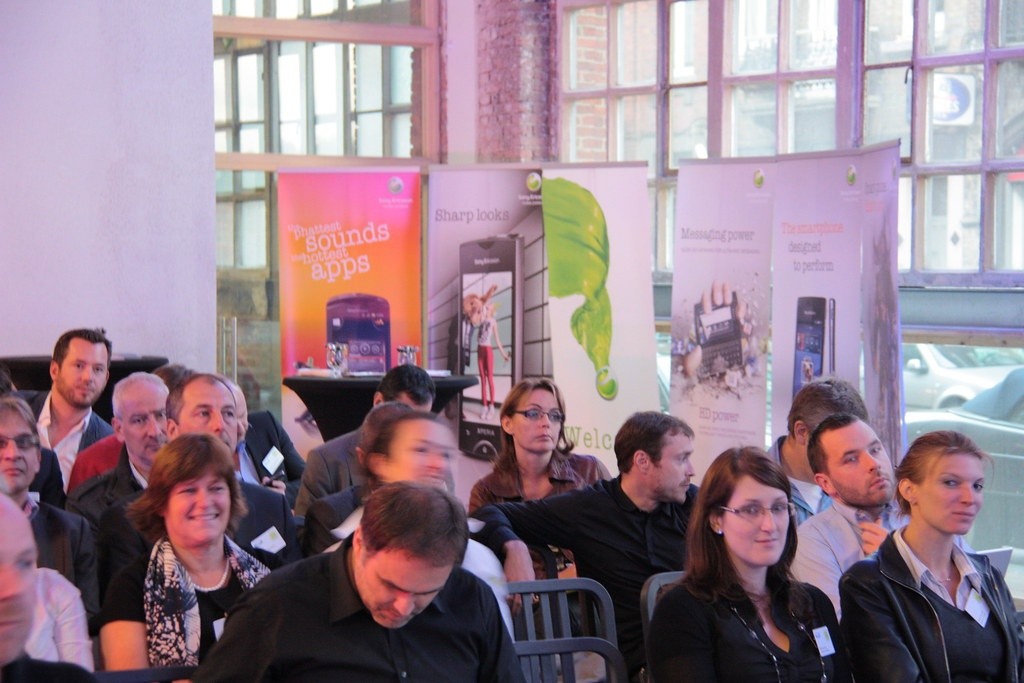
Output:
[282,373,481,441]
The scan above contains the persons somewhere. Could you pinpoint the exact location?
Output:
[469,376,612,593]
[189,480,525,683]
[787,413,975,622]
[0,396,98,671]
[476,303,509,420]
[767,378,869,524]
[0,368,66,507]
[66,364,201,492]
[839,431,1024,683]
[646,447,854,683]
[99,433,272,669]
[0,493,97,683]
[469,411,700,683]
[98,373,306,596]
[685,279,752,380]
[294,364,437,520]
[66,372,170,539]
[304,401,515,640]
[18,328,115,489]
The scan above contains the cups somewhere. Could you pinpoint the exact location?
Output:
[397,345,418,367]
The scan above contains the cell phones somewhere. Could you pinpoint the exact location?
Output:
[456,233,522,457]
[326,292,391,373]
[792,297,835,396]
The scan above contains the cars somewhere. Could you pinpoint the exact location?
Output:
[901,343,1024,416]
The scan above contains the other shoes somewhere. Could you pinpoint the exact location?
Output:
[486,404,495,420]
[481,405,489,419]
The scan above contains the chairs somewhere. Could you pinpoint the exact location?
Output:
[501,570,687,683]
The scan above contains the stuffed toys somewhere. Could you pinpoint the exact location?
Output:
[462,294,493,366]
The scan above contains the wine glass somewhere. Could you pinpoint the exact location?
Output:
[326,344,347,378]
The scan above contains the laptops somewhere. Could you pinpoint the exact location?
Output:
[694,291,745,378]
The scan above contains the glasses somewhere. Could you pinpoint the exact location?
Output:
[0,435,39,449]
[514,408,562,422]
[718,505,790,521]
